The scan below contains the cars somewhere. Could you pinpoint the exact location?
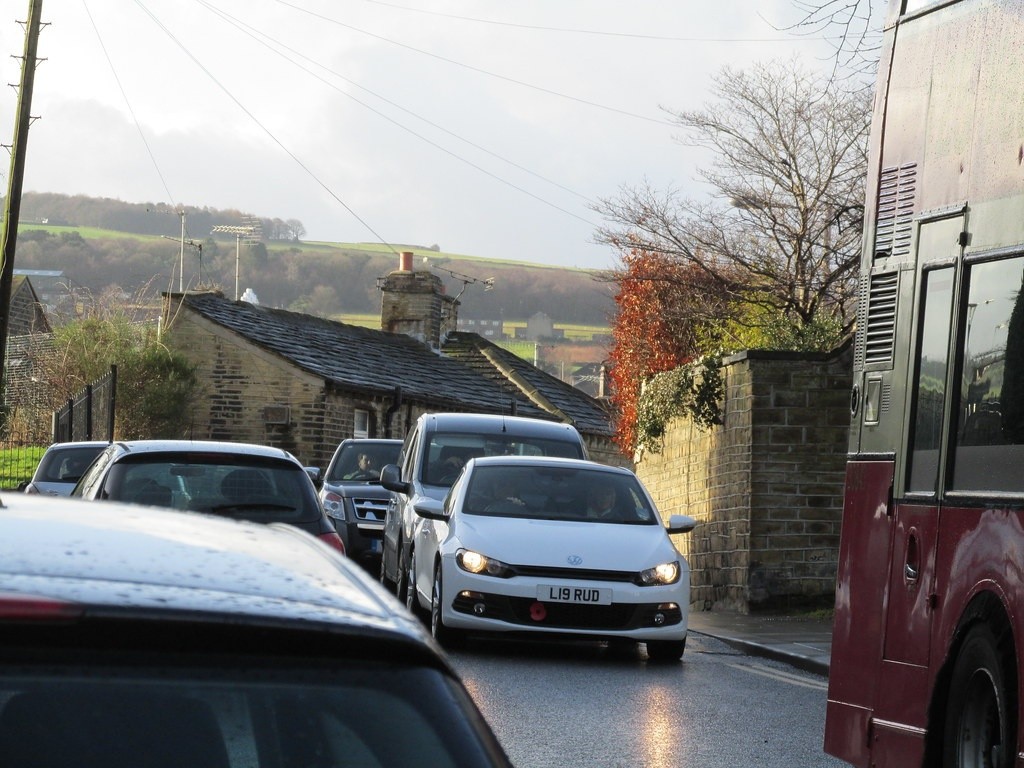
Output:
[66,438,345,556]
[0,493,514,768]
[378,413,590,602]
[403,455,697,660]
[302,437,404,559]
[22,443,193,511]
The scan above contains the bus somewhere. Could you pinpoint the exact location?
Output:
[822,0,1024,768]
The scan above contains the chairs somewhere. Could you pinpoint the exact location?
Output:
[124,478,165,508]
[219,468,272,504]
[438,445,485,463]
[0,690,229,768]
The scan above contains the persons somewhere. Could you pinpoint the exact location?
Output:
[472,475,531,513]
[581,481,624,521]
[442,449,486,476]
[341,451,381,479]
